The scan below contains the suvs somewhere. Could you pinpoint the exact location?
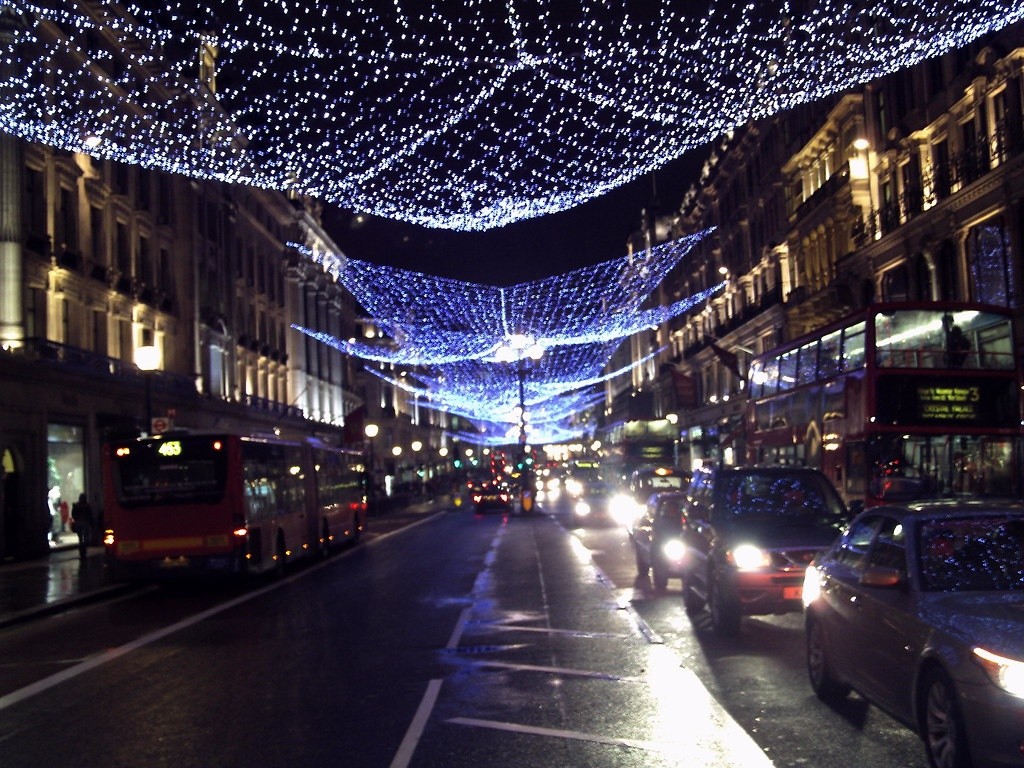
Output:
[677,467,851,637]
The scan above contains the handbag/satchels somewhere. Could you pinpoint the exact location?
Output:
[72,522,78,532]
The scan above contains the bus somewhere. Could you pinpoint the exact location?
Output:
[101,436,367,591]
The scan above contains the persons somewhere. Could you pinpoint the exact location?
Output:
[48,486,62,542]
[778,480,816,514]
[72,494,92,566]
[920,527,976,591]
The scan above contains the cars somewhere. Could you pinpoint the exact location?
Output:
[628,492,688,591]
[475,484,514,516]
[799,497,1023,767]
[537,462,610,526]
